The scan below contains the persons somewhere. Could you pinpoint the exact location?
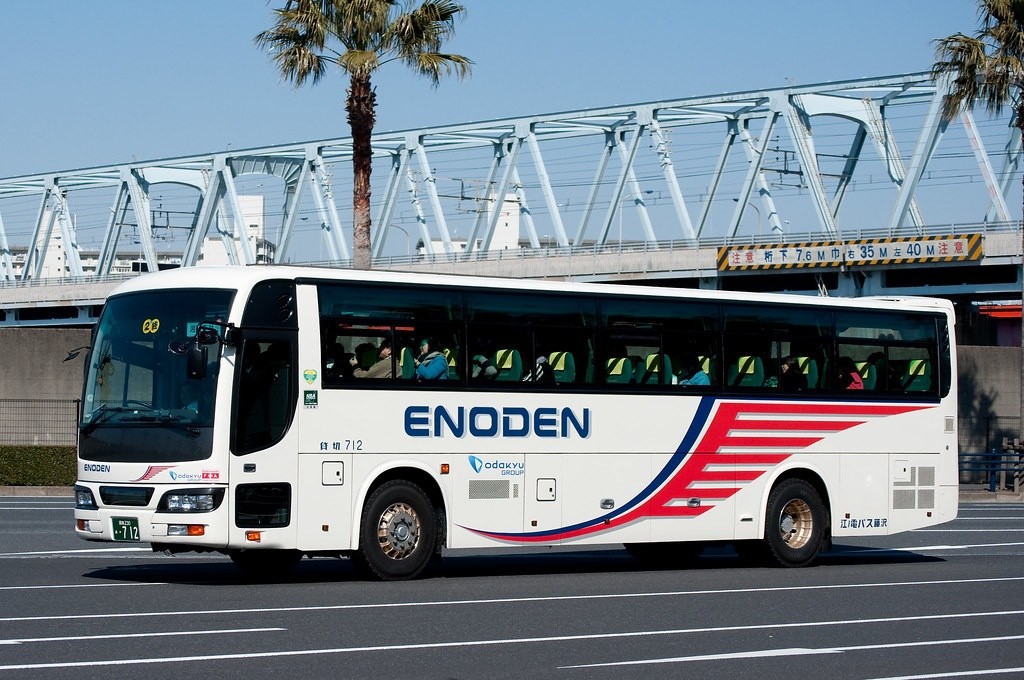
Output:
[413,335,449,380]
[166,381,199,413]
[867,352,902,392]
[836,356,864,390]
[778,355,808,391]
[471,342,498,379]
[678,356,710,386]
[523,346,555,383]
[326,338,402,378]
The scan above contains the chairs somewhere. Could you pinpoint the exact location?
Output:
[549,351,575,382]
[736,355,764,386]
[855,361,876,389]
[643,353,672,384]
[903,359,931,392]
[494,349,522,381]
[400,347,414,377]
[795,356,818,388]
[606,357,631,383]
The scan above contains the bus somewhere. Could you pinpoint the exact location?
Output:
[62,267,959,581]
